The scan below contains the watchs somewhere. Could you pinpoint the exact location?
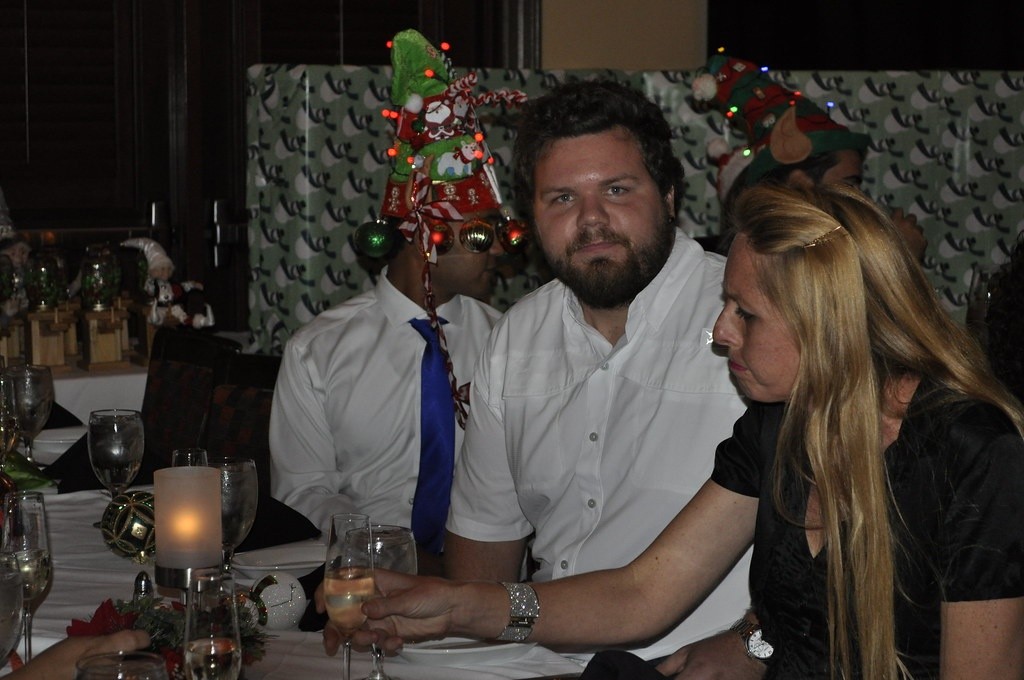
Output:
[730,619,774,664]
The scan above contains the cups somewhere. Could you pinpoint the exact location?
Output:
[183,568,243,680]
[74,650,169,680]
[0,375,19,467]
[153,465,223,598]
[0,552,23,669]
[171,448,208,468]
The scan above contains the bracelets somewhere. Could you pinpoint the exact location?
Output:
[494,582,539,643]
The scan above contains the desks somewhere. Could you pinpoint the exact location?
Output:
[0,484,589,680]
[25,359,151,428]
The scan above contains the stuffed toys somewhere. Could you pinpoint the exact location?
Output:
[144,258,219,329]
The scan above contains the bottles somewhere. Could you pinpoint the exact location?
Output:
[133,570,154,612]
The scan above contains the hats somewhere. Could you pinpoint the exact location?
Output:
[353,28,529,261]
[707,138,764,205]
[693,54,871,172]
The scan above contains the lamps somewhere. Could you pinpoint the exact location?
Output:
[152,467,226,600]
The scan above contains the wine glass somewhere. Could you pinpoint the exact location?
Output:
[210,457,259,568]
[6,364,54,463]
[323,514,374,680]
[342,525,418,680]
[88,408,145,528]
[2,491,54,665]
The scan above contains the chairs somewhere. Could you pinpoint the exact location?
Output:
[83,324,320,563]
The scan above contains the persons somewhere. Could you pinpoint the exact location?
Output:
[265,30,528,582]
[443,83,776,680]
[314,182,1024,679]
[694,54,930,263]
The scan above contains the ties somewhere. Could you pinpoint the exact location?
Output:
[406,316,457,575]
